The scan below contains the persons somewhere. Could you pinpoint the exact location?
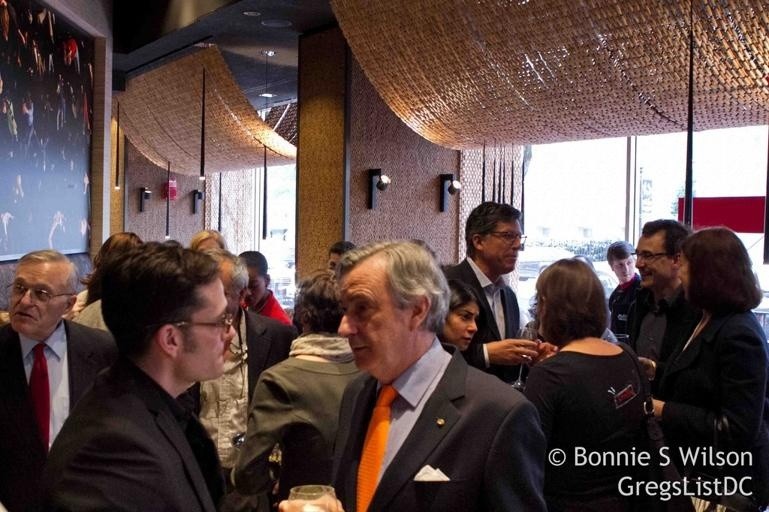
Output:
[574,253,619,345]
[0,247,118,511]
[623,219,699,403]
[607,241,643,341]
[43,238,238,510]
[275,236,548,511]
[517,253,692,512]
[633,226,766,511]
[446,201,560,389]
[525,263,547,326]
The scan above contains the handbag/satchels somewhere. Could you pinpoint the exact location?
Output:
[631,416,699,512]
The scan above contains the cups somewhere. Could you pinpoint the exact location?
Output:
[289,483,340,512]
[606,333,630,345]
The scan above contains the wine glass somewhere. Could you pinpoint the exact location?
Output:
[509,327,538,387]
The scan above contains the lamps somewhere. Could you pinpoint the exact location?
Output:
[139,187,152,212]
[438,172,463,213]
[192,189,205,214]
[366,167,392,210]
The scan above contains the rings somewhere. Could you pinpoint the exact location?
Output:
[522,353,530,362]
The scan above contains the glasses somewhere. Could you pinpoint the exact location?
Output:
[6,282,74,302]
[488,231,527,246]
[629,252,676,261]
[172,312,234,334]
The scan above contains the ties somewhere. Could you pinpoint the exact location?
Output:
[28,344,51,454]
[353,385,403,512]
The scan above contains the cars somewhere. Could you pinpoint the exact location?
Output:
[264,242,298,318]
[526,270,620,317]
[516,250,571,282]
